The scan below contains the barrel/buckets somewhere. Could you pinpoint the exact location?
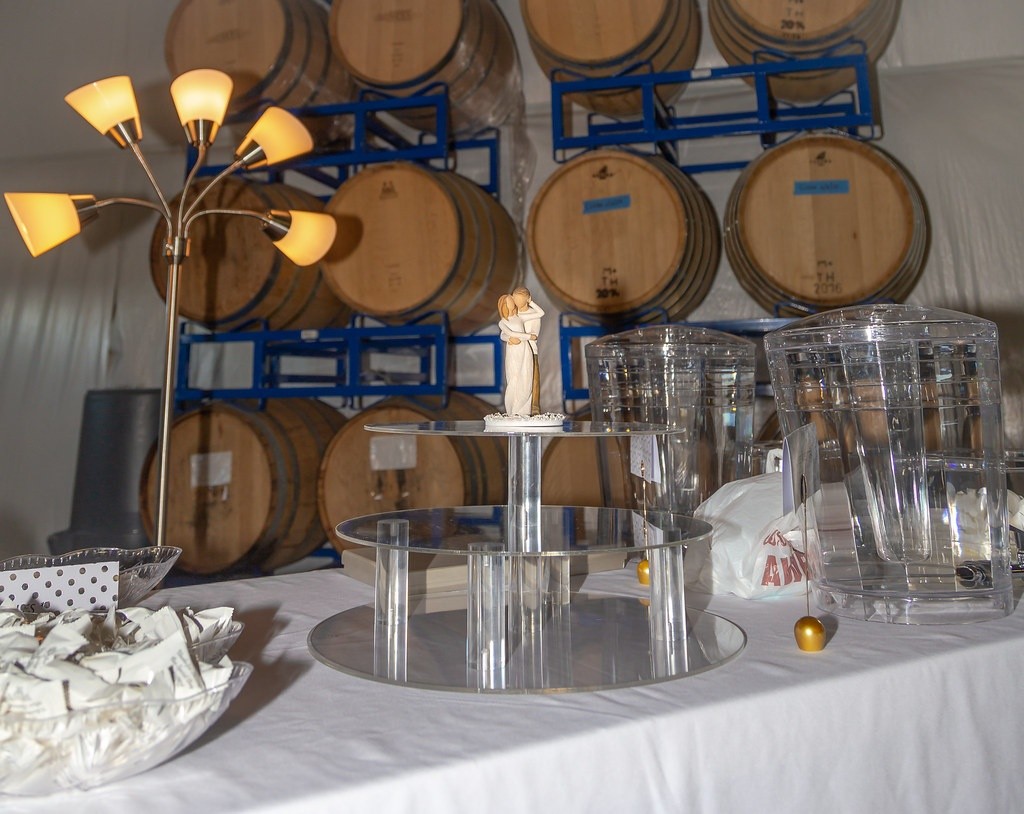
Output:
[328,1,523,138]
[760,374,985,483]
[151,178,354,328]
[138,394,349,576]
[524,146,720,323]
[707,0,900,101]
[319,388,508,557]
[163,1,374,145]
[543,397,735,517]
[320,164,530,335]
[519,1,702,115]
[724,131,929,318]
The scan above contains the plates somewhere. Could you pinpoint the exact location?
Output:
[0,544,182,608]
[186,620,244,664]
[0,662,253,796]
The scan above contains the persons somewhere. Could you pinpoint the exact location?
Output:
[498,287,545,419]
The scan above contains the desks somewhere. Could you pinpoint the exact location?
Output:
[1,549,1023,814]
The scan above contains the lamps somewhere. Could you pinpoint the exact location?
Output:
[4,76,338,589]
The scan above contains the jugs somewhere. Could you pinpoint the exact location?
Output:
[584,326,757,523]
[764,302,1016,626]
